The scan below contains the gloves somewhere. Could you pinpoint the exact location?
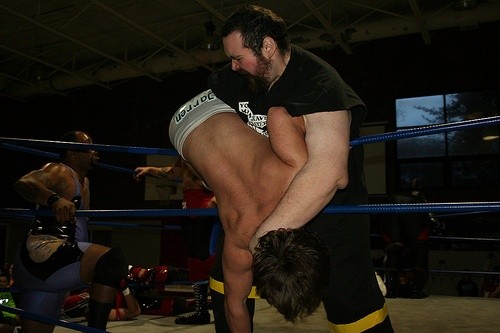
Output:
[116,275,131,291]
[130,264,154,285]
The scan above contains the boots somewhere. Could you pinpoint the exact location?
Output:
[175,295,214,313]
[175,280,211,324]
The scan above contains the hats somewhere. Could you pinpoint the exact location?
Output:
[63,293,89,317]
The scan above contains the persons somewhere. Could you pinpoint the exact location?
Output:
[0,131,142,333]
[134,3,396,333]
